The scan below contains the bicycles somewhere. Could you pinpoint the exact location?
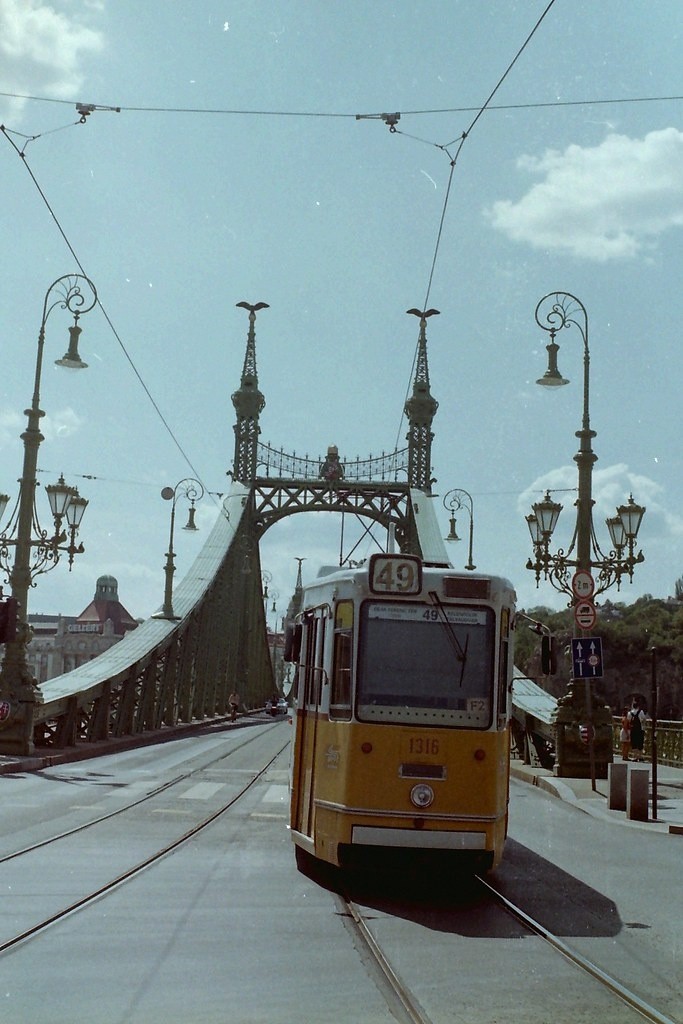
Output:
[229,702,239,723]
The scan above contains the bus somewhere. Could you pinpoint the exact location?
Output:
[276,553,559,876]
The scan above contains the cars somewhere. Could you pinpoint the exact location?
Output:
[264,698,289,714]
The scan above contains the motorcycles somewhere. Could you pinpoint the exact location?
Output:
[270,699,280,717]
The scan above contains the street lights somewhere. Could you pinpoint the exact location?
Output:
[444,488,477,571]
[261,570,273,599]
[527,291,644,792]
[0,273,97,757]
[150,478,204,620]
[273,610,285,679]
[265,590,279,616]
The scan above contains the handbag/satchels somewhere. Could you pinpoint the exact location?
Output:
[620,729,631,741]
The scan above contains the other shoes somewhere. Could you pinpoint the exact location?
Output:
[631,759,638,762]
[623,756,625,760]
[639,758,644,761]
[625,758,631,761]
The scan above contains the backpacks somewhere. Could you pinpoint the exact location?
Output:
[629,710,641,731]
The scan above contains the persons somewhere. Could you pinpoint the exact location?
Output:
[228,688,240,722]
[627,702,646,762]
[269,692,279,706]
[619,710,631,761]
